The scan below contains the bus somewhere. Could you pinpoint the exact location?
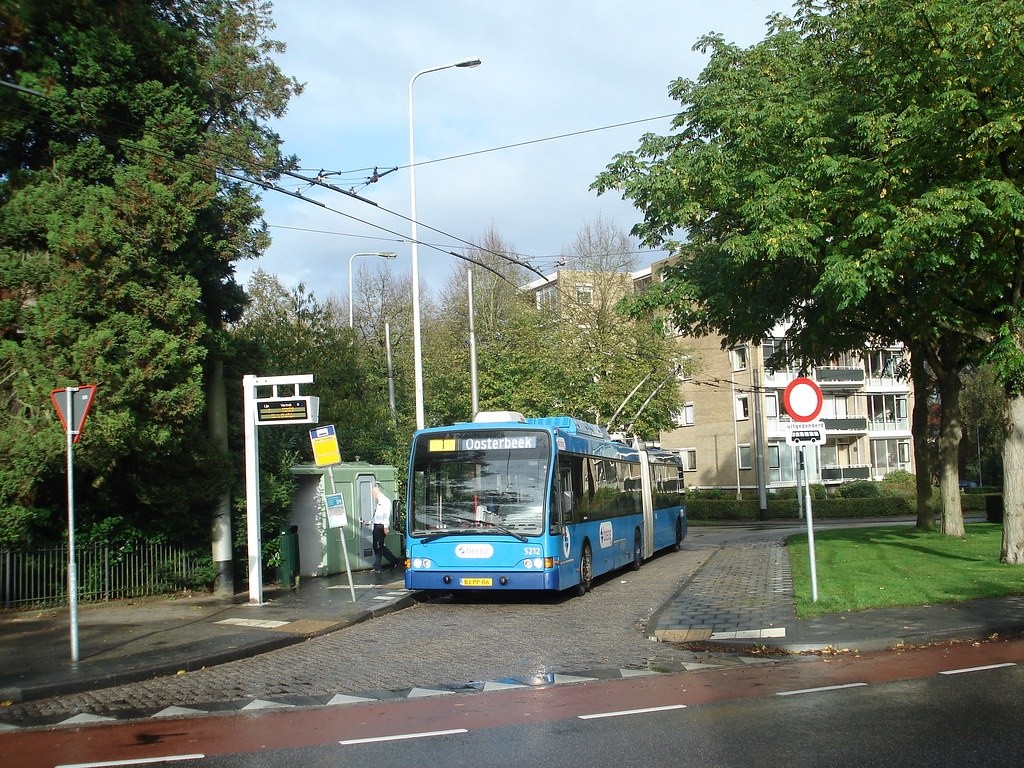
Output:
[404,411,687,596]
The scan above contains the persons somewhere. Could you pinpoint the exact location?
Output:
[365,487,401,573]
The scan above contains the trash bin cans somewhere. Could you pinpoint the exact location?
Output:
[274,525,301,587]
[985,493,1004,523]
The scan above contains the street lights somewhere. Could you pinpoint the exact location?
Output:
[345,251,397,342]
[407,57,484,431]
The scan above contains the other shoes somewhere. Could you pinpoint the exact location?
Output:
[389,559,402,572]
[369,568,382,575]
